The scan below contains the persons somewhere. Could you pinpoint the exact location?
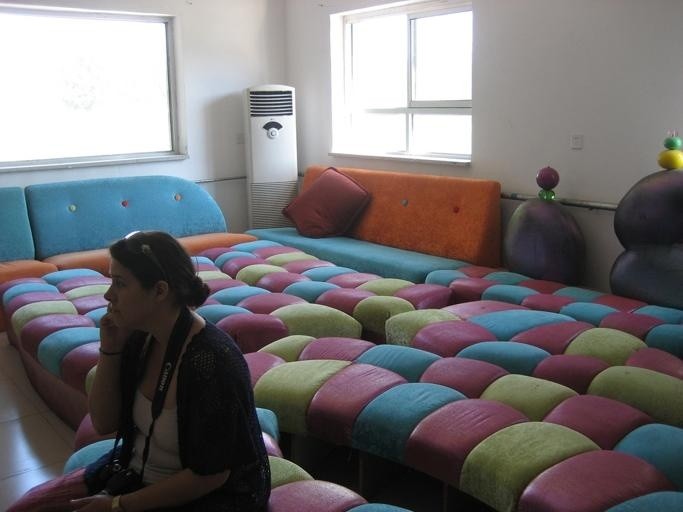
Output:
[5,230,271,512]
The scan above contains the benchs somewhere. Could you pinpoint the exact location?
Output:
[0,165,682,511]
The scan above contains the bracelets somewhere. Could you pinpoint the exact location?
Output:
[99,347,125,356]
[111,495,124,512]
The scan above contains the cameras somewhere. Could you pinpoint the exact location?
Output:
[98,461,146,496]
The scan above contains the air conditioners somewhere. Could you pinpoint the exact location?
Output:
[242,83,300,231]
